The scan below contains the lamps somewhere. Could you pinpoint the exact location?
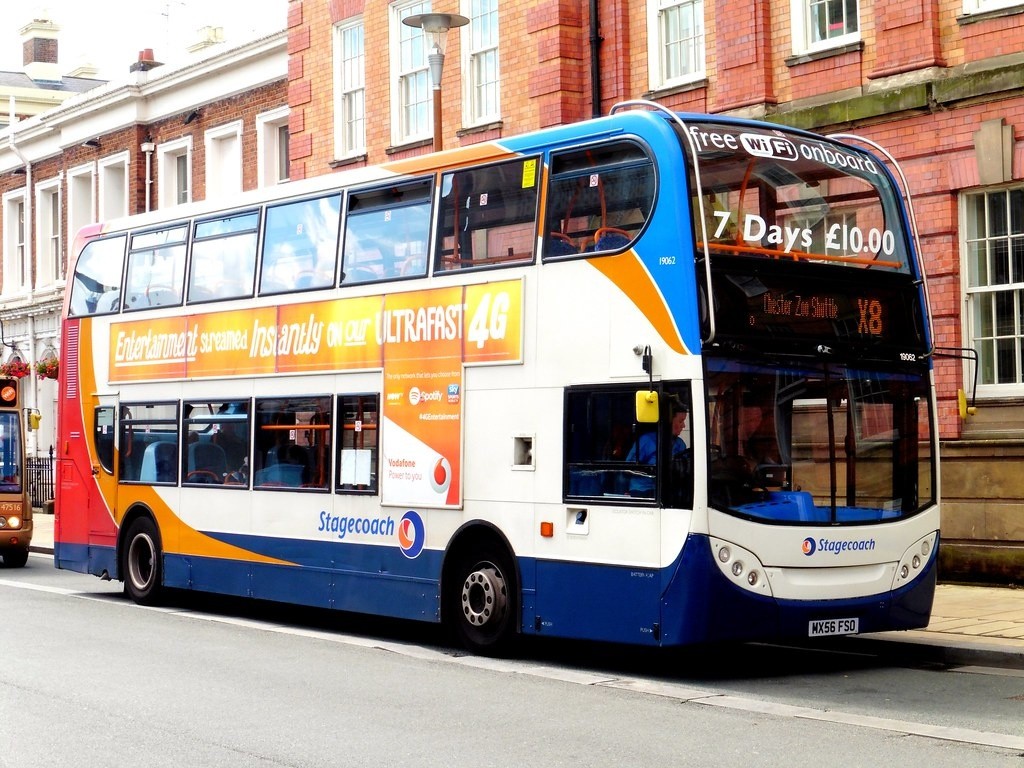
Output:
[12,170,30,177]
[183,112,201,125]
[81,141,102,148]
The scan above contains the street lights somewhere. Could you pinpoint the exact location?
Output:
[402,12,471,154]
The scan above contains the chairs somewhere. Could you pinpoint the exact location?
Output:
[124,228,633,313]
[140,441,367,492]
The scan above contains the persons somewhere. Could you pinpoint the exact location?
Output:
[738,408,769,491]
[188,422,242,447]
[622,404,687,501]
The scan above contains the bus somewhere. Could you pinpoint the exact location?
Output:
[0,375,42,572]
[51,98,981,662]
